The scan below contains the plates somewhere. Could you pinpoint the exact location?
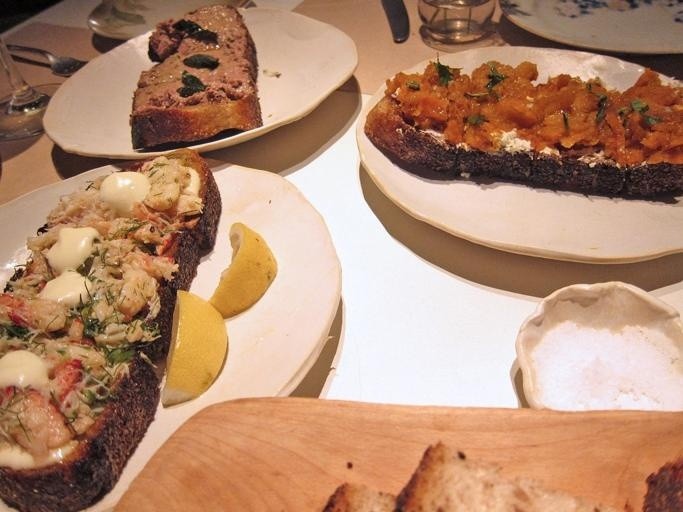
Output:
[0,153,341,511]
[498,0,682,58]
[39,10,360,159]
[87,2,307,42]
[359,42,682,265]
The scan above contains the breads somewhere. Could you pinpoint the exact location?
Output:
[0,149,220,512]
[322,481,398,512]
[363,60,683,205]
[640,454,683,512]
[397,441,615,512]
[130,3,263,148]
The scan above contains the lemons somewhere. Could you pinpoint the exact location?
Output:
[161,288,228,407]
[209,223,277,319]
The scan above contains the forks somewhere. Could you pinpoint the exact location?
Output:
[7,43,88,76]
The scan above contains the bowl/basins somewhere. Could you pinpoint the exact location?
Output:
[514,282,682,410]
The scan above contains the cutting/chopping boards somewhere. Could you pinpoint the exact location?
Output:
[106,395,683,512]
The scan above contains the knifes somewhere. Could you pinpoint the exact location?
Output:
[380,0,411,42]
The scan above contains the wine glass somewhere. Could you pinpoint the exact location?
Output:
[0,22,62,140]
